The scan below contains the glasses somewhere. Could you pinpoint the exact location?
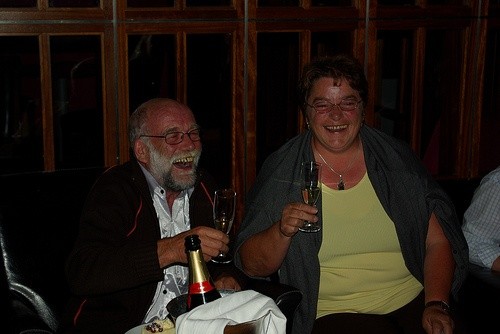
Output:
[305,99,364,114]
[137,128,204,145]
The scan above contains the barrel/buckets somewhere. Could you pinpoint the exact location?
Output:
[167,289,271,334]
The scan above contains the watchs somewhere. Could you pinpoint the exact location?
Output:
[424,300,450,313]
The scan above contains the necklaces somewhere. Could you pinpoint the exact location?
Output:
[313,139,361,190]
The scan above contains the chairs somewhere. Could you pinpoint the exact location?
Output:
[0,168,304,334]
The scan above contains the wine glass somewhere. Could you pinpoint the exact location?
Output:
[297,162,324,232]
[211,189,237,263]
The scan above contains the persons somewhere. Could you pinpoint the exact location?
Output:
[230,54,469,334]
[461,166,500,272]
[57,98,241,334]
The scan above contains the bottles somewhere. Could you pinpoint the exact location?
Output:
[184,234,222,312]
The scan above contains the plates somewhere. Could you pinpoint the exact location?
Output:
[125,323,153,334]
